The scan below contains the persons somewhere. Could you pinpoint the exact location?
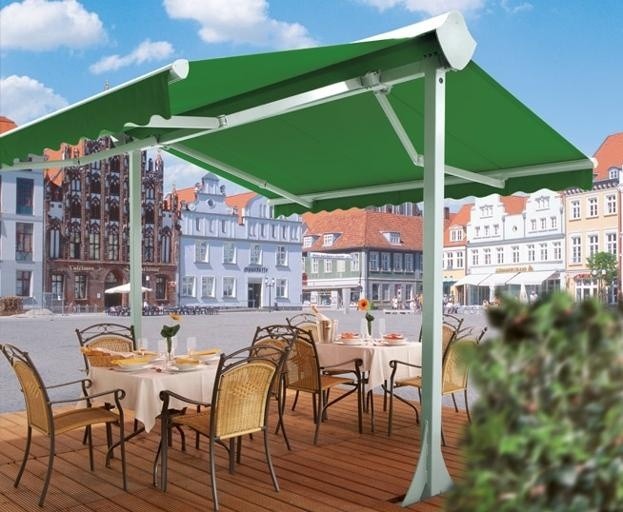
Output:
[389,290,538,314]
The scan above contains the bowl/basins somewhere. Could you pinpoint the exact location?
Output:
[383,336,407,343]
[174,363,193,369]
[119,362,147,370]
[341,338,361,343]
[200,353,217,361]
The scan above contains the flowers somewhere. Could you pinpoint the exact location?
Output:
[359,299,373,334]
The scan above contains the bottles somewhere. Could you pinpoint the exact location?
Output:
[311,305,329,321]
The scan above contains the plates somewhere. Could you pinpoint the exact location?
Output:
[168,367,199,372]
[336,341,364,346]
[382,342,408,346]
[113,364,152,373]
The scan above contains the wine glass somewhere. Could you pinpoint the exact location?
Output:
[360,318,366,339]
[138,337,148,357]
[379,318,385,345]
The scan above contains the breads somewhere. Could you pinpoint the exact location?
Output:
[200,349,217,357]
[109,357,147,365]
[174,357,200,366]
[139,350,159,356]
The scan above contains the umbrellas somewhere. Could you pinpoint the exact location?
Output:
[104,280,153,305]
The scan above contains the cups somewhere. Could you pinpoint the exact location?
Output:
[186,336,197,360]
